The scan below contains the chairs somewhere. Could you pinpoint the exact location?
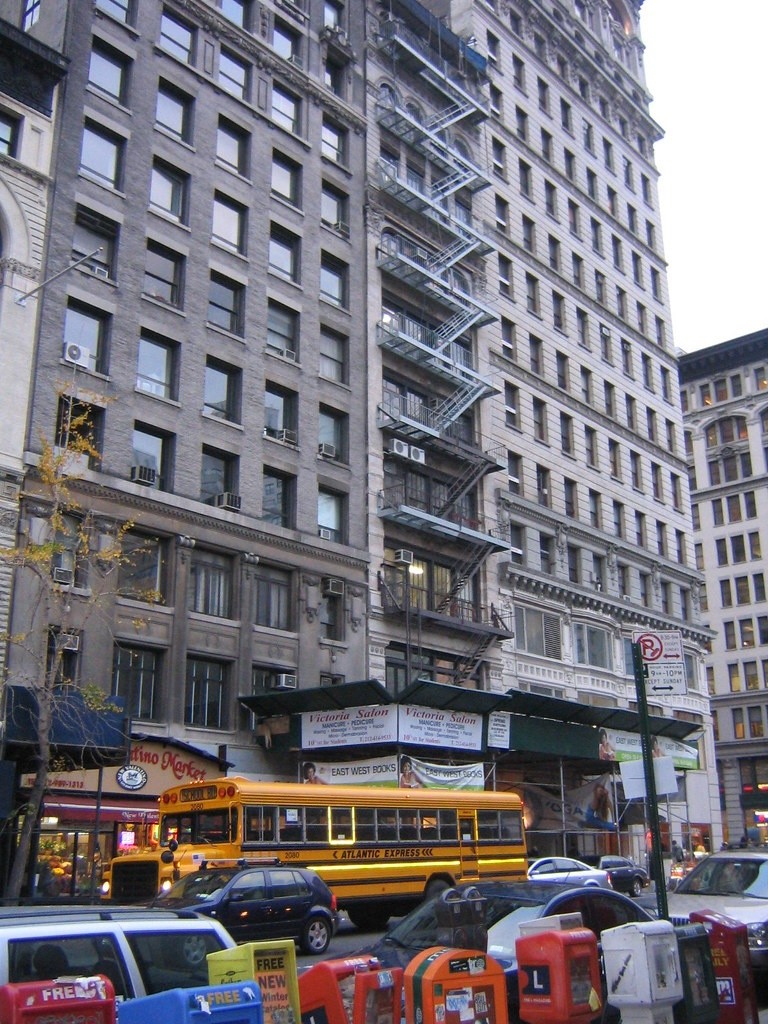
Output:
[33,945,69,979]
[93,944,125,996]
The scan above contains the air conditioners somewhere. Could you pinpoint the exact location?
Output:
[318,529,331,540]
[394,549,413,565]
[382,12,395,23]
[92,267,109,278]
[275,674,296,690]
[387,438,425,464]
[276,429,297,444]
[63,342,89,368]
[619,595,631,602]
[56,634,79,651]
[279,348,296,361]
[214,492,242,512]
[131,465,156,487]
[334,26,347,39]
[334,221,350,238]
[50,567,74,585]
[622,343,629,351]
[287,55,303,69]
[324,579,345,596]
[319,442,336,458]
[411,247,427,262]
[600,327,610,336]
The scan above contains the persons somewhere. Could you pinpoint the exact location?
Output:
[568,840,583,860]
[578,783,616,830]
[600,729,615,760]
[400,756,423,790]
[650,735,664,761]
[671,836,748,862]
[527,847,540,868]
[303,763,324,785]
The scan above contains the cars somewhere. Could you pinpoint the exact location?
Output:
[644,852,768,975]
[580,855,650,898]
[524,857,613,901]
[298,883,668,1024]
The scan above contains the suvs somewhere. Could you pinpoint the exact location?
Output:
[0,904,242,1024]
[128,857,340,954]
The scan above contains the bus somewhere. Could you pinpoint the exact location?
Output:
[101,775,528,927]
